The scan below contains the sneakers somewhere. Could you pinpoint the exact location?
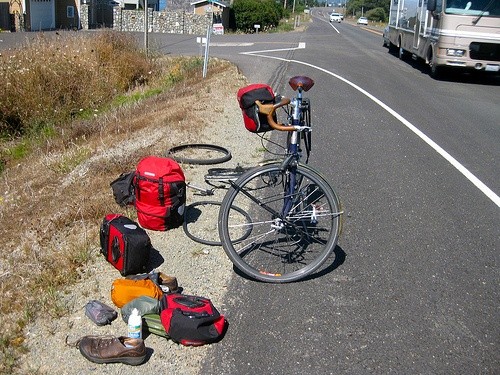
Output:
[78,335,147,365]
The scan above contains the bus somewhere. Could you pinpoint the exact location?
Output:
[389,0,500,82]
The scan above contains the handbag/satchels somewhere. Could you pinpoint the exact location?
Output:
[112,269,182,306]
[134,153,187,230]
[237,83,278,133]
[159,293,227,347]
[99,212,150,275]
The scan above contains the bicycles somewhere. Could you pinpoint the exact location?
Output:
[218,76,346,284]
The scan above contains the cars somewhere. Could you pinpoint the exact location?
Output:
[339,13,344,20]
[382,26,389,47]
[356,17,368,25]
[329,12,341,23]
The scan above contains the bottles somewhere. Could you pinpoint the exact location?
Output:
[127,307,143,339]
